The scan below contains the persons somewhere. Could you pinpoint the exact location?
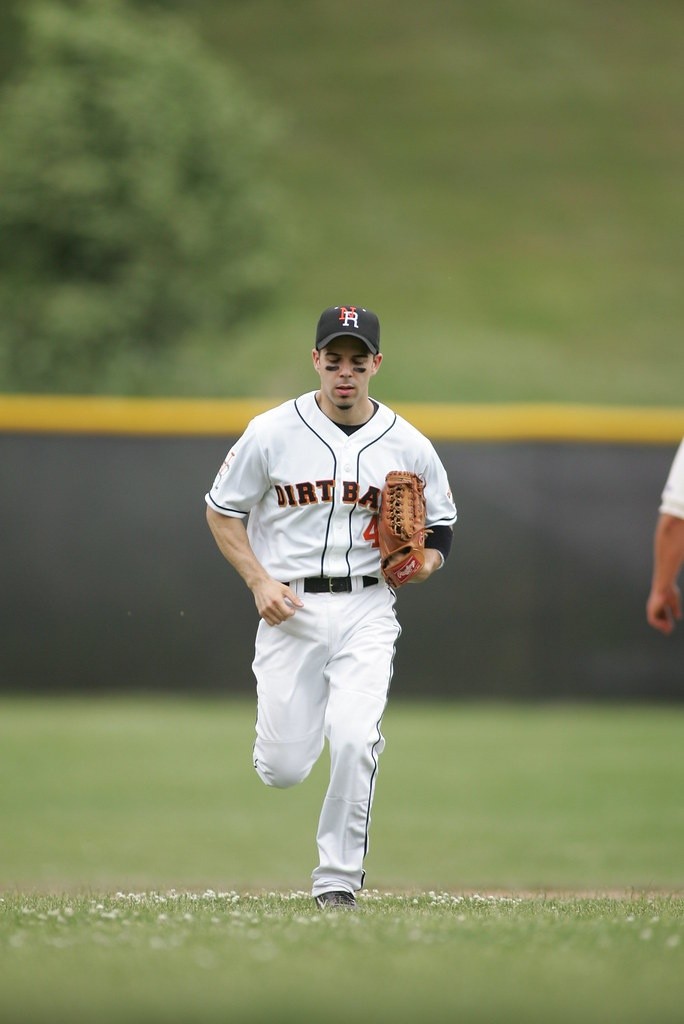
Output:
[645,436,684,634]
[203,306,458,912]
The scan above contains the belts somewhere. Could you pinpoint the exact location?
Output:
[282,575,378,594]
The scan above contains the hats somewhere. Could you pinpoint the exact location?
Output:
[316,306,380,355]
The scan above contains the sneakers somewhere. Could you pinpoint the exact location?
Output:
[315,891,357,912]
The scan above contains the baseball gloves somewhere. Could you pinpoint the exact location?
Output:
[377,470,428,589]
